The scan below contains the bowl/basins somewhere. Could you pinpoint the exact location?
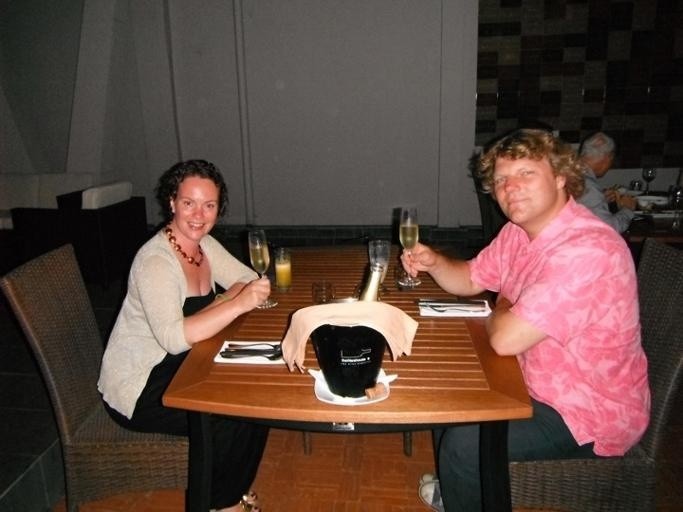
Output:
[636,195,669,208]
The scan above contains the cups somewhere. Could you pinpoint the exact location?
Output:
[311,280,336,306]
[275,247,292,288]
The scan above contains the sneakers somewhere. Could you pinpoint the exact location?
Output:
[417,472,444,512]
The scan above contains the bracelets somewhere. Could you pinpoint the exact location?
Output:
[214,292,227,302]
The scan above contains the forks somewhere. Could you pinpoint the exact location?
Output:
[425,302,487,313]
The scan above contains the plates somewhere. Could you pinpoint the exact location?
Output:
[313,366,392,406]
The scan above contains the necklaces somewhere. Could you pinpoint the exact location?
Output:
[167,226,206,269]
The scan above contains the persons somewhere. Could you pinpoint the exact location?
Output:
[94,159,272,512]
[401,126,653,511]
[568,129,638,237]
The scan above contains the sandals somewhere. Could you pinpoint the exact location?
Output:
[209,487,262,512]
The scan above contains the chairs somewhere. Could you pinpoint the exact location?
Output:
[10,181,149,280]
[0,241,190,512]
[509,237,683,512]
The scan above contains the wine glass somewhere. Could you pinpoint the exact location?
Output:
[642,165,657,196]
[397,207,422,286]
[368,239,391,296]
[247,229,278,310]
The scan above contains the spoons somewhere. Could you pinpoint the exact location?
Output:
[220,343,283,361]
